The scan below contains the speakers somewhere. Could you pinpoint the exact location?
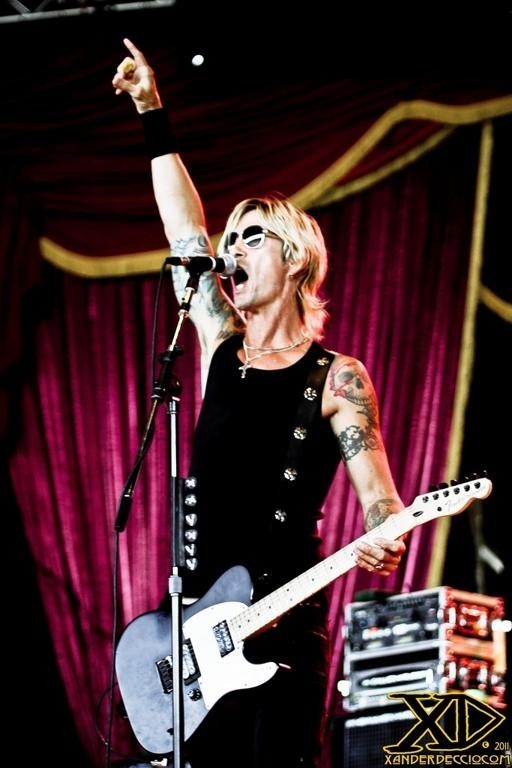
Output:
[345,708,504,767]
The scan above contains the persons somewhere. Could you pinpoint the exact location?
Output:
[95,33,414,767]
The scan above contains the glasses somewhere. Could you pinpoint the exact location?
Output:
[224,225,281,253]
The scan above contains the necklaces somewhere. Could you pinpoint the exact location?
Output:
[233,336,311,379]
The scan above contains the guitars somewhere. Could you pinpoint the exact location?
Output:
[115,471,494,755]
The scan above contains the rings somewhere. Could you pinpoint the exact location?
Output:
[375,563,385,571]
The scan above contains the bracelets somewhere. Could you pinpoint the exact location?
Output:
[134,105,181,161]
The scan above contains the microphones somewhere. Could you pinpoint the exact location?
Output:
[166,253,237,278]
